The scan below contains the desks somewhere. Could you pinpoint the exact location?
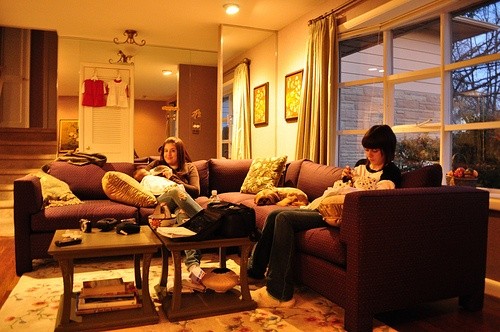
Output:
[48,225,162,332]
[153,228,260,324]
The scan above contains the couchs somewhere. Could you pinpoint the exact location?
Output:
[12,158,490,332]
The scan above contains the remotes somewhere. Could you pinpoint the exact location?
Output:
[55,237,82,247]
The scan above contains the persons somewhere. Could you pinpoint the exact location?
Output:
[239,125,399,307]
[134,136,201,270]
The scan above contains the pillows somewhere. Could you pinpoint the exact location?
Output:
[140,175,185,195]
[239,156,288,193]
[254,187,309,206]
[101,171,157,207]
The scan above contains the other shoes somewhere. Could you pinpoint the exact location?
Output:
[238,286,295,307]
[238,277,265,284]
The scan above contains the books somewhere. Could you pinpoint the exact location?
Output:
[156,226,198,238]
[74,278,143,315]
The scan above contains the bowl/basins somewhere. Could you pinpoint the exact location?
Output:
[149,213,176,229]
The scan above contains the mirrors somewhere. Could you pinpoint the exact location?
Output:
[217,24,279,159]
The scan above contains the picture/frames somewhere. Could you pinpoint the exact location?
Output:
[59,119,79,152]
[284,68,303,121]
[253,82,269,126]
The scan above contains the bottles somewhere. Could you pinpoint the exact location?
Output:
[209,190,220,202]
[342,166,353,187]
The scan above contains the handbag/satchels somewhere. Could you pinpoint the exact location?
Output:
[170,201,259,242]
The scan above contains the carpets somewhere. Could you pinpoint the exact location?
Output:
[0,258,398,332]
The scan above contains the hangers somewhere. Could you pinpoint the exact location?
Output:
[107,74,128,85]
[84,72,104,83]
